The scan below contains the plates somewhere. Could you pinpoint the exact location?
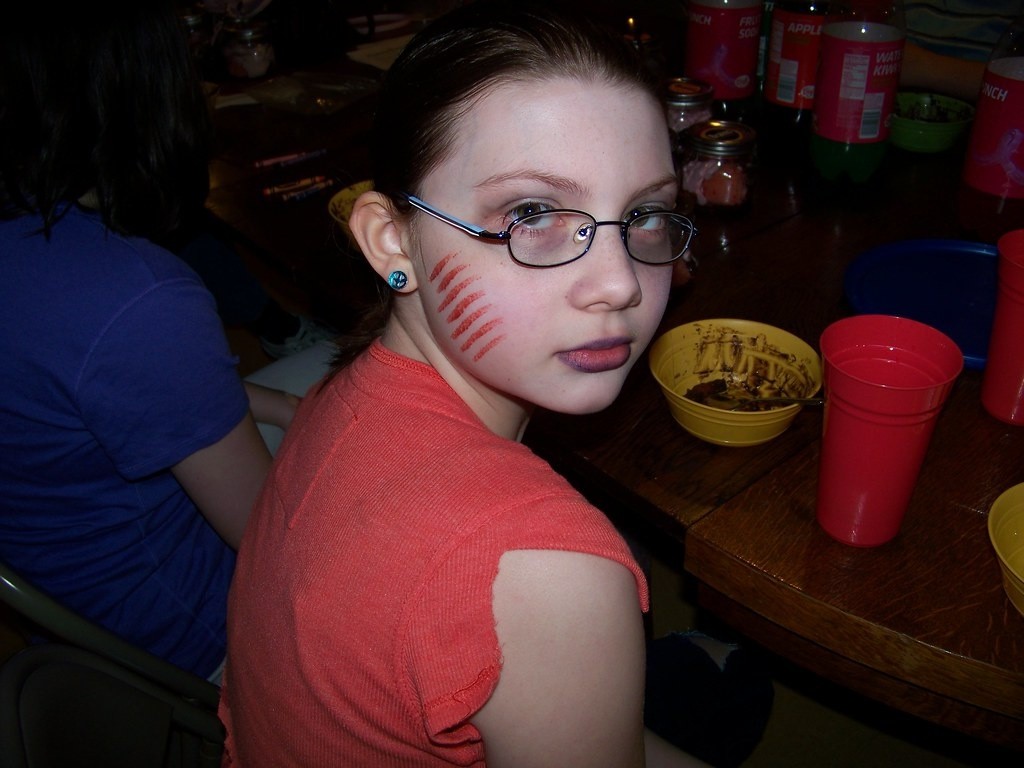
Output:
[843,240,997,370]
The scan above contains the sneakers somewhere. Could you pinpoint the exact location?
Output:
[259,312,354,367]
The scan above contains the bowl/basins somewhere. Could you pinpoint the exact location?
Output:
[648,319,822,447]
[987,481,1024,617]
[890,92,975,152]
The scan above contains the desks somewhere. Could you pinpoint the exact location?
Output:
[526,54,1024,748]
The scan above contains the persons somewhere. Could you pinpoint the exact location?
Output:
[0,0,369,692]
[215,0,776,767]
[890,0,1024,97]
[162,0,369,370]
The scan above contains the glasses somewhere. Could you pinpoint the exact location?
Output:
[391,189,702,268]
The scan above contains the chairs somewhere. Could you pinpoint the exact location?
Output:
[0,565,226,768]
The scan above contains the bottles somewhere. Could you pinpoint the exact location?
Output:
[800,0,907,214]
[684,0,762,181]
[949,0,1024,237]
[661,76,715,167]
[682,120,769,224]
[744,0,827,188]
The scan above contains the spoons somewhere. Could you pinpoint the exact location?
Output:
[705,394,824,411]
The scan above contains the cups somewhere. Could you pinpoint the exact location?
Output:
[813,314,963,547]
[979,229,1024,427]
